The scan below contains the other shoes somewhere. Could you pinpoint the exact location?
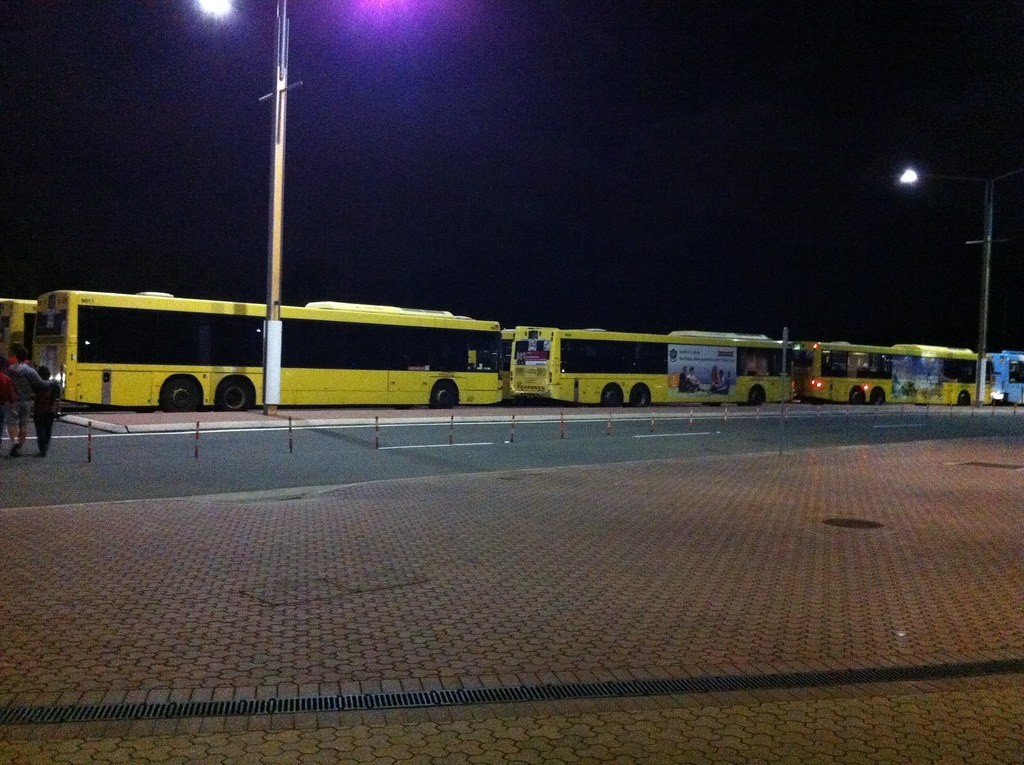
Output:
[8,442,23,456]
[41,449,47,457]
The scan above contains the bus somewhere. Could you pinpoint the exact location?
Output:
[500,329,517,399]
[32,290,503,412]
[0,299,38,389]
[795,341,994,405]
[511,325,810,407]
[989,350,1024,404]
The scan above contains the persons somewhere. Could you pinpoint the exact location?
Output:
[679,366,703,392]
[0,342,66,457]
[708,366,731,394]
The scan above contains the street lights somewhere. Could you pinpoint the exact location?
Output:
[199,1,396,413]
[898,168,1022,355]
